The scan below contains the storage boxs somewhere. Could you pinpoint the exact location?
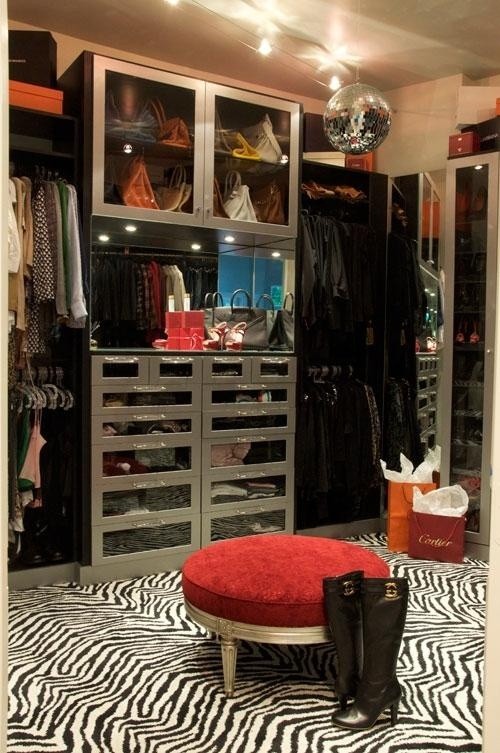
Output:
[166,310,205,350]
[8,28,65,114]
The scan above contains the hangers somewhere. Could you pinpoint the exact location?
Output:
[92,245,218,274]
[10,365,74,414]
[312,363,355,404]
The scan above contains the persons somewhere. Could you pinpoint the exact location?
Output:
[339,108,349,132]
[353,103,387,135]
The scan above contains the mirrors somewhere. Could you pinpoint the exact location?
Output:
[386,169,446,472]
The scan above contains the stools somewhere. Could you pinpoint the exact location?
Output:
[181,533,393,699]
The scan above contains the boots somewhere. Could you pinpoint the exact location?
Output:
[322,571,364,710]
[331,577,409,731]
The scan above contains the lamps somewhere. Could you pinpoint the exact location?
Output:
[271,251,281,257]
[223,236,235,243]
[124,225,137,232]
[191,243,201,250]
[98,234,110,242]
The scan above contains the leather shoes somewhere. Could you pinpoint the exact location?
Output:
[451,186,487,445]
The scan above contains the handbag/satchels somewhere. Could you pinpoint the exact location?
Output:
[409,512,465,564]
[214,120,285,224]
[200,307,293,350]
[106,111,192,213]
[387,481,436,553]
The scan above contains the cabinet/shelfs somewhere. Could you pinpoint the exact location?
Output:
[58,354,203,386]
[57,49,303,238]
[58,386,296,585]
[446,151,499,546]
[203,355,297,384]
[59,216,303,353]
